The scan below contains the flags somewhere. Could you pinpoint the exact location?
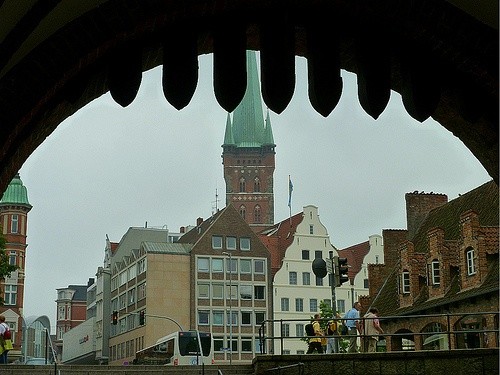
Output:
[288,179,294,208]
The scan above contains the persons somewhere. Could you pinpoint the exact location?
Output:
[325,311,342,354]
[0,314,11,364]
[342,302,361,353]
[361,308,384,353]
[305,313,324,354]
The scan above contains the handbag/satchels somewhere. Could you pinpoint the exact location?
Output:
[341,326,348,335]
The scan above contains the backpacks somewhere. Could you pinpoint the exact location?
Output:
[305,322,318,336]
[326,322,334,335]
[1,324,11,339]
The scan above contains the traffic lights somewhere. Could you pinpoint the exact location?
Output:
[140,311,144,325]
[334,256,349,287]
[113,310,118,325]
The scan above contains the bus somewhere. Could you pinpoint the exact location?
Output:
[133,329,214,367]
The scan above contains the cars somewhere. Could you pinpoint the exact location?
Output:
[24,357,51,365]
